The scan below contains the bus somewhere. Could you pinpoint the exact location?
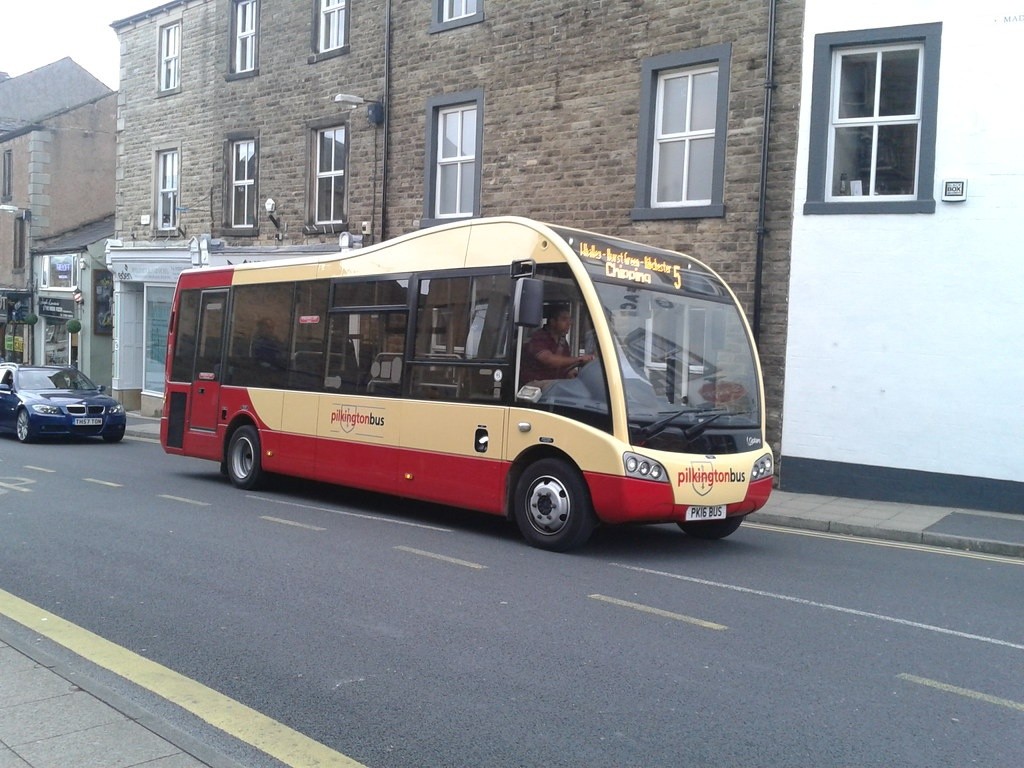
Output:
[161,212,774,555]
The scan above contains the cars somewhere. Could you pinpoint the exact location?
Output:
[0,362,127,444]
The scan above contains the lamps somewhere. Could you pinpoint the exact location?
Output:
[334,93,386,108]
[73,287,85,305]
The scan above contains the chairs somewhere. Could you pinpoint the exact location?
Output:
[178,332,380,395]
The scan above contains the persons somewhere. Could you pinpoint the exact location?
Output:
[521,307,597,389]
